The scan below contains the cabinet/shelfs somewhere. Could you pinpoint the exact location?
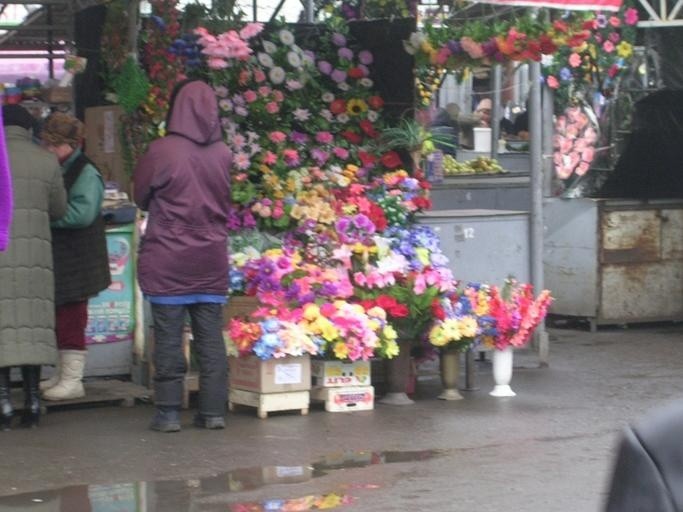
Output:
[542,196,683,332]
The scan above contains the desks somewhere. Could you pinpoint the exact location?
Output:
[6,224,133,383]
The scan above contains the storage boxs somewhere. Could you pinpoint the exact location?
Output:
[85,105,131,201]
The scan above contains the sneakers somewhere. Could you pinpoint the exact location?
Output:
[149,410,179,433]
[194,413,224,428]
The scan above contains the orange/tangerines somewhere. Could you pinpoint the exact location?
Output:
[442,154,503,174]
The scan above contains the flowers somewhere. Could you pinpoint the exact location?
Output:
[100,0,640,418]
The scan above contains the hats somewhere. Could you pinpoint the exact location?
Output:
[38,109,83,144]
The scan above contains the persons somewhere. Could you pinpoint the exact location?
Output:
[36,111,111,402]
[0,105,67,427]
[433,91,528,148]
[133,81,229,431]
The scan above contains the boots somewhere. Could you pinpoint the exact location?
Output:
[20,364,41,425]
[0,367,13,426]
[40,348,87,401]
